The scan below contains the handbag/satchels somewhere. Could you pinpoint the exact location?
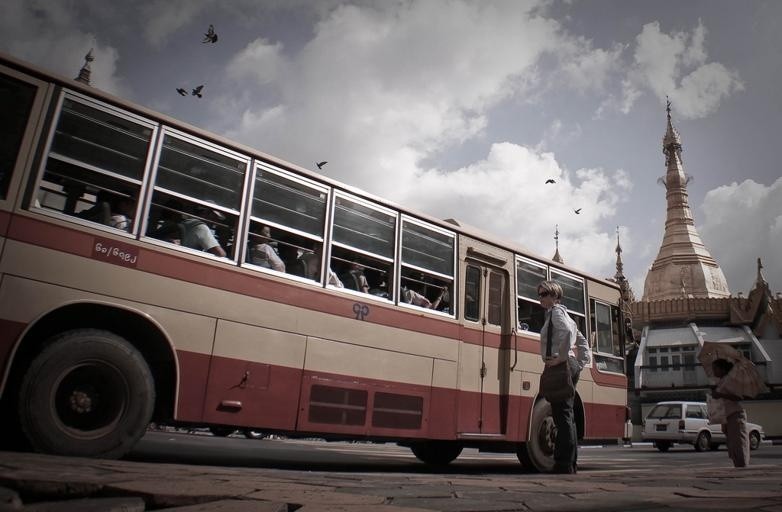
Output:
[540,361,574,403]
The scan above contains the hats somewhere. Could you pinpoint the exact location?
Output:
[198,200,226,221]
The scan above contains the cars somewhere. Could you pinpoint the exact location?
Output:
[638,400,767,453]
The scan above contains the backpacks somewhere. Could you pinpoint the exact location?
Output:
[176,219,205,248]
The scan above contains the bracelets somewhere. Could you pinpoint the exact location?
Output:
[437,295,442,301]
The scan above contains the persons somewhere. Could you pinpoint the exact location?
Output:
[350,253,372,294]
[247,218,286,275]
[307,232,346,289]
[408,275,448,310]
[174,192,229,258]
[401,285,431,307]
[98,174,133,233]
[708,358,750,469]
[535,277,591,479]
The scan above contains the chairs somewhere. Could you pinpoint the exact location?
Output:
[76,201,409,303]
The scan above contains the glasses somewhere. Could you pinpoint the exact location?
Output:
[538,292,548,298]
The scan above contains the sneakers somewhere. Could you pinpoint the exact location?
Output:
[542,466,560,474]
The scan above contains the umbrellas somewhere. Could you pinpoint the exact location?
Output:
[696,339,762,402]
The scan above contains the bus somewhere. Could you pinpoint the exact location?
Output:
[2,49,639,474]
